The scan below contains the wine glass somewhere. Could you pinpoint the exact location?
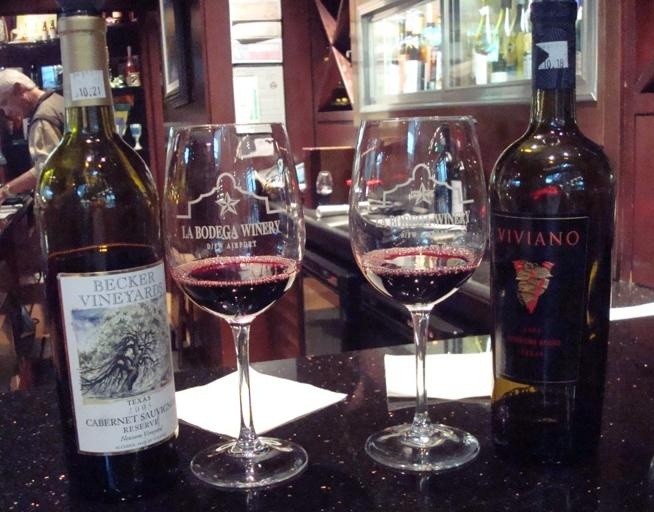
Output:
[316,172,333,208]
[130,123,142,151]
[164,121,309,489]
[349,115,489,473]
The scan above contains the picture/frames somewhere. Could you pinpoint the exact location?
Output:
[158,0,191,109]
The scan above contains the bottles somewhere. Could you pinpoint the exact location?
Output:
[30,8,183,493]
[390,3,489,91]
[492,1,618,453]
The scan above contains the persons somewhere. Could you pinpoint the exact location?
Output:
[0,68,95,260]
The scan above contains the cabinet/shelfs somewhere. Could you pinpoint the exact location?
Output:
[0,19,148,184]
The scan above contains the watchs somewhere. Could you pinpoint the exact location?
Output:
[2,183,17,199]
